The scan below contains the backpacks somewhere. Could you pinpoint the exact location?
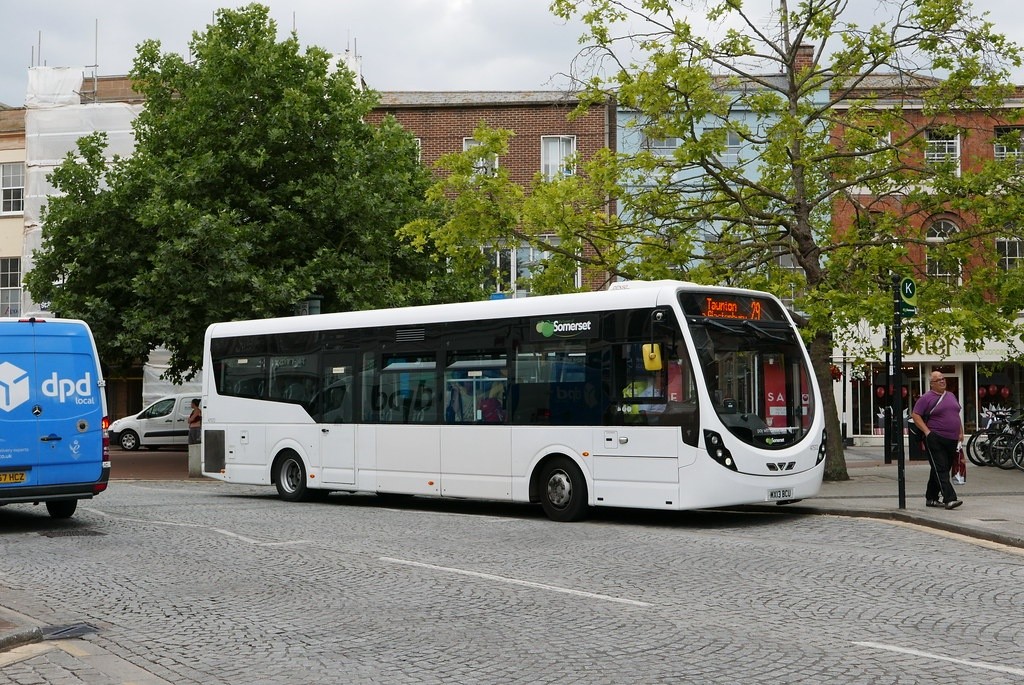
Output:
[479,389,505,423]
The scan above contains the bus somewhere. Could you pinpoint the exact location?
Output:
[201,280,824,522]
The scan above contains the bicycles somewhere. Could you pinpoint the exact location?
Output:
[967,408,1024,470]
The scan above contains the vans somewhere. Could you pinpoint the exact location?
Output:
[108,394,201,452]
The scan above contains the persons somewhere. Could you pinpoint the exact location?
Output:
[912,371,964,510]
[637,374,668,424]
[188,398,202,444]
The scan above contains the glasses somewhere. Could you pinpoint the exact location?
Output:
[931,378,945,382]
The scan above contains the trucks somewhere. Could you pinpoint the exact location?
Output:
[0,316,112,519]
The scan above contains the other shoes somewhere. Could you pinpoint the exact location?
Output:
[926,499,963,509]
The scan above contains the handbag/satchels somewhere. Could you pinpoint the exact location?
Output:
[446,406,456,424]
[916,412,930,442]
[951,440,967,486]
